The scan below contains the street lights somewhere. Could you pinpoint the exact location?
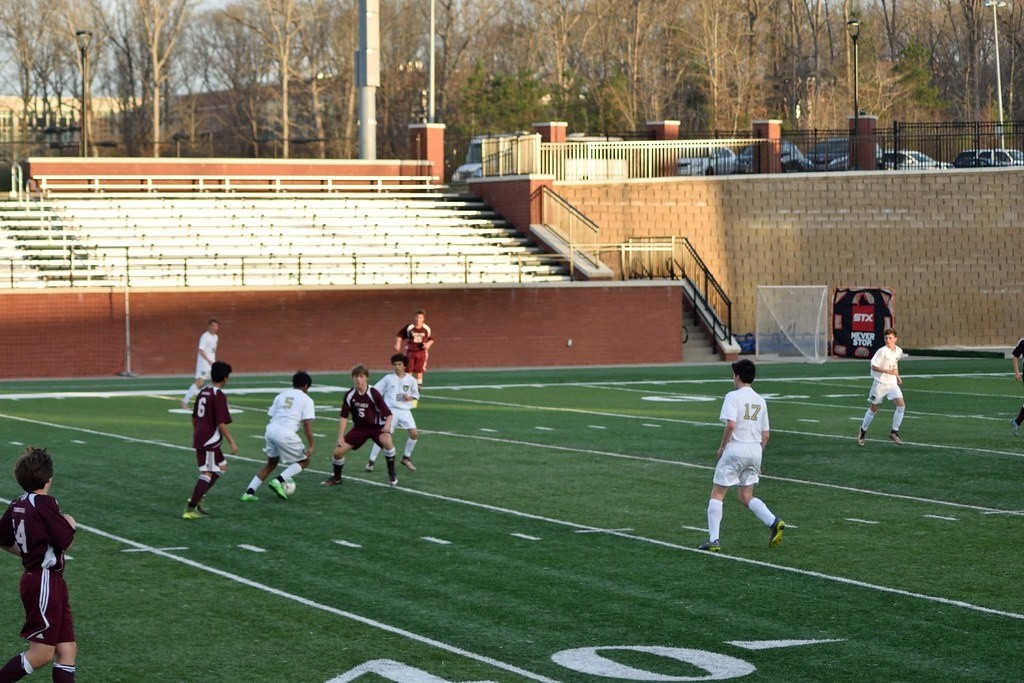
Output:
[846,18,865,168]
[984,0,1006,148]
[75,30,94,157]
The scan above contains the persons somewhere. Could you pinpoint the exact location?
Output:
[365,354,420,471]
[240,372,315,501]
[183,362,238,519]
[1012,339,1024,436]
[179,319,218,412]
[394,310,435,389]
[0,449,76,683]
[320,365,396,486]
[697,359,784,551]
[857,328,905,446]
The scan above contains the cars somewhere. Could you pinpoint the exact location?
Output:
[677,146,738,176]
[800,136,883,172]
[734,139,803,174]
[881,149,1024,171]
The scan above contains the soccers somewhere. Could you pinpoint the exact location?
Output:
[282,477,296,495]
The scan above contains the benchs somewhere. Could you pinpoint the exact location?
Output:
[0,191,574,290]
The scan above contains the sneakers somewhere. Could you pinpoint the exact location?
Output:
[240,493,259,501]
[1012,418,1020,437]
[889,431,903,445]
[696,538,721,552]
[365,461,375,472]
[269,479,289,499]
[858,425,866,446]
[390,477,398,488]
[400,457,417,472]
[320,476,342,486]
[768,518,786,548]
[182,499,209,519]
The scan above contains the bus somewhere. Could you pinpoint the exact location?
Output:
[451,130,629,184]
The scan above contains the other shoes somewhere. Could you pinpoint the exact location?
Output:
[180,402,190,411]
[414,398,418,410]
